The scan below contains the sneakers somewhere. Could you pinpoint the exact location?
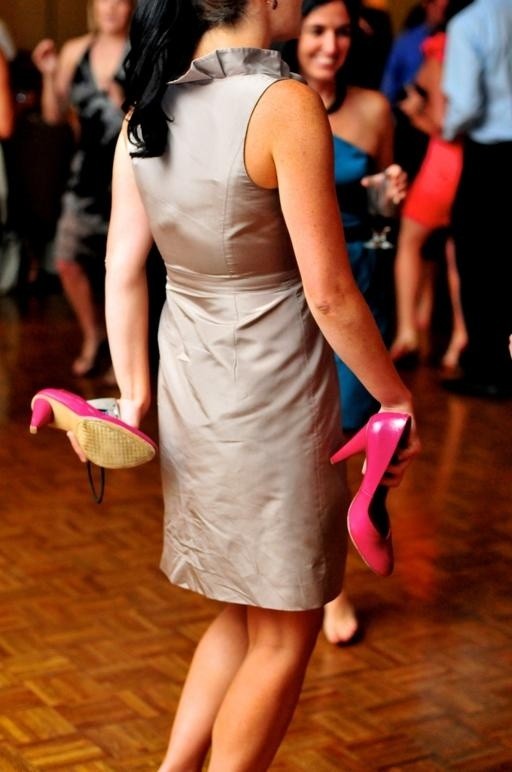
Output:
[435,367,511,404]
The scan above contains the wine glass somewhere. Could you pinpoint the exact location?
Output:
[362,180,394,251]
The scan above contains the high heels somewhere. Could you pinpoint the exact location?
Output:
[319,410,412,581]
[23,382,158,474]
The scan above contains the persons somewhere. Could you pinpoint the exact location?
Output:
[68,0,422,770]
[277,0,412,647]
